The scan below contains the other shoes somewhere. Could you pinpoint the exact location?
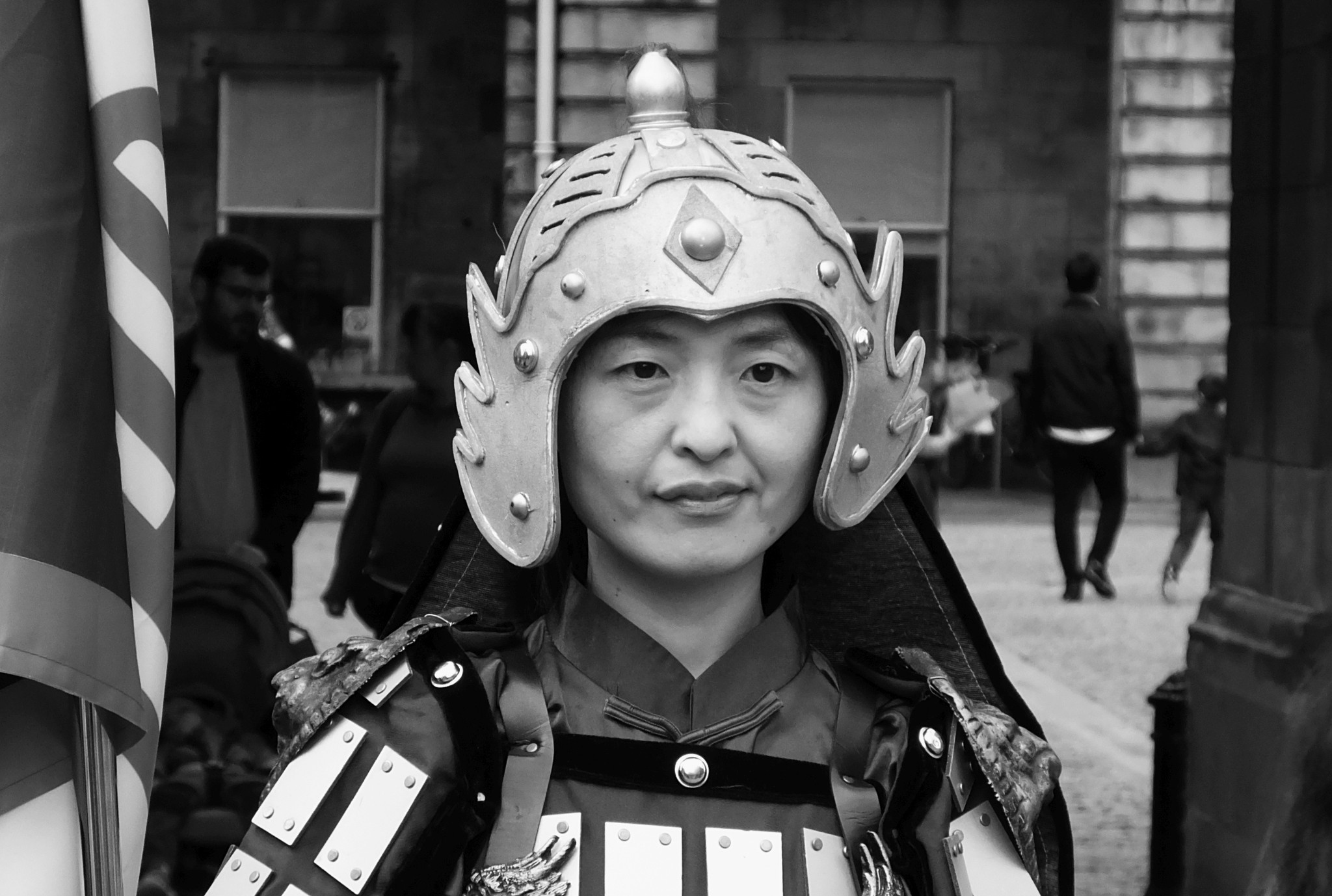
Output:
[1162,563,1179,604]
[1062,559,1116,599]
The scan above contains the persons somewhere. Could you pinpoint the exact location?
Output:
[1133,371,1229,601]
[169,239,325,607]
[324,301,480,639]
[1020,252,1142,602]
[200,53,1074,894]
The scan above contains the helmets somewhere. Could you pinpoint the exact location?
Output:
[451,51,935,569]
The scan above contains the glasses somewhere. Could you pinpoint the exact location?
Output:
[218,283,268,303]
